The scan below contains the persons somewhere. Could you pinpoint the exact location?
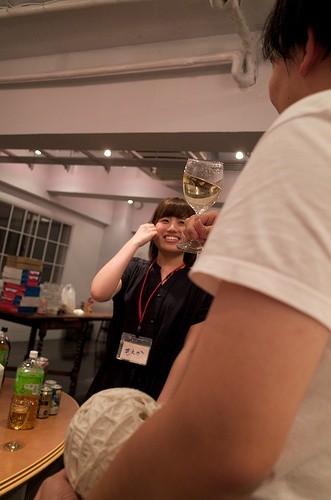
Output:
[81,198,211,406]
[34,1,331,499]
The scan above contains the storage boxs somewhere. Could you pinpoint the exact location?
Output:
[0,256,44,313]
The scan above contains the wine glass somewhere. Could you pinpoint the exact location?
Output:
[176,158,224,255]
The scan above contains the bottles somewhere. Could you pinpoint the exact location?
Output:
[7,350,45,431]
[0,326,11,383]
[86,297,93,313]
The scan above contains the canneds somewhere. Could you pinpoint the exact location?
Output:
[36,379,62,418]
[37,299,47,314]
[87,297,93,313]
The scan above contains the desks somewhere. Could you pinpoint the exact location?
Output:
[0,309,114,398]
[0,375,80,500]
